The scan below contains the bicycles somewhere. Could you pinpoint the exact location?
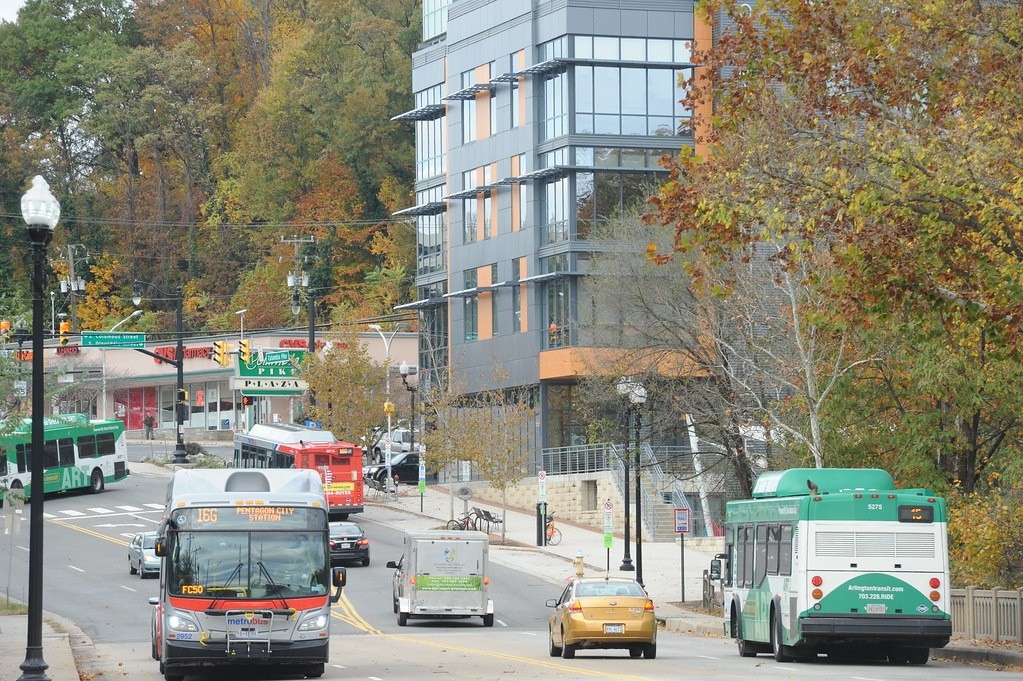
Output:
[546,511,562,545]
[446,510,477,530]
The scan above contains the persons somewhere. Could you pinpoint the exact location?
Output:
[143,412,154,440]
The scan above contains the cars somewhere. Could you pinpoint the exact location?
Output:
[362,418,437,485]
[129,532,162,578]
[545,576,658,660]
[329,522,369,567]
[149,597,161,661]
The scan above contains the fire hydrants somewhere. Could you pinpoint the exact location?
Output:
[573,551,585,576]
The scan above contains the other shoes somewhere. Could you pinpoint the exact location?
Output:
[151,438,155,440]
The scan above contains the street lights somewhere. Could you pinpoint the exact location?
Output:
[290,290,316,418]
[17,174,61,681]
[368,324,404,489]
[615,375,648,596]
[132,280,189,464]
[103,309,143,420]
[234,309,248,431]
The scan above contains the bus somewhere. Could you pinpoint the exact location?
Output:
[710,467,952,661]
[232,421,365,521]
[156,467,348,681]
[0,412,136,506]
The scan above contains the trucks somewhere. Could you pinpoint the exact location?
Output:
[386,529,494,628]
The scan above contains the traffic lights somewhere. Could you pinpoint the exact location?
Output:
[60,321,69,346]
[239,339,249,363]
[0,321,10,343]
[177,391,187,401]
[214,341,234,367]
[242,396,253,404]
